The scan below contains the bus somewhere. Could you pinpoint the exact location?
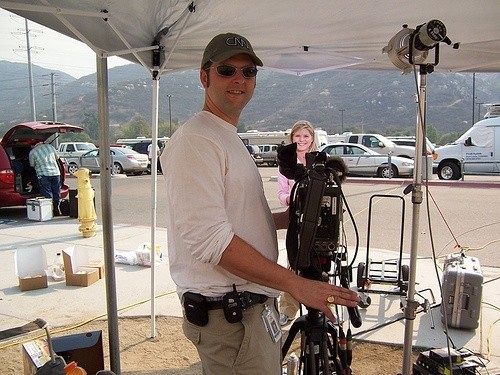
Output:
[117,128,327,148]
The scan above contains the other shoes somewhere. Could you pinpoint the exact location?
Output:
[55,209,62,215]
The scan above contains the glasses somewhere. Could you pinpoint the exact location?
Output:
[203,64,259,79]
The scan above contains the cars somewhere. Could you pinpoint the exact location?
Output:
[54,142,170,175]
[385,136,434,154]
[318,143,415,181]
[0,121,86,208]
[66,147,148,176]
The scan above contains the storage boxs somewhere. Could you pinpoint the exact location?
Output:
[80,260,105,279]
[14,245,49,291]
[27,198,53,221]
[136,245,163,266]
[62,245,100,287]
[22,338,57,375]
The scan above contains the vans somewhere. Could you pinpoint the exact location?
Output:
[257,144,279,167]
[431,117,500,181]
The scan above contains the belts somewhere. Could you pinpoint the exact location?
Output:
[204,300,225,311]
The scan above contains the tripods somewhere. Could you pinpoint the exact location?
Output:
[281,252,361,375]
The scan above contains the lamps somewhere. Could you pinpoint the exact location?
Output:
[383,19,451,75]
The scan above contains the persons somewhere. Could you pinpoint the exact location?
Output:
[276,119,320,206]
[28,138,62,216]
[147,141,160,160]
[159,33,360,375]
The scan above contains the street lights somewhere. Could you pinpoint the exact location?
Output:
[166,94,173,138]
[339,109,346,134]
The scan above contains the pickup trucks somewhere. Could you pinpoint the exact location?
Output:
[344,133,415,159]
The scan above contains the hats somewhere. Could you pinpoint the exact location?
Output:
[201,33,263,68]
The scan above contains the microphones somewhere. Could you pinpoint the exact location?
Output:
[325,156,347,185]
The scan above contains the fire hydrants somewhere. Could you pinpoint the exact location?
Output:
[73,167,98,238]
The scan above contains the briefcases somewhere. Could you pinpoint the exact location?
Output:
[26,196,54,221]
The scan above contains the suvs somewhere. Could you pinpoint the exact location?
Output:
[245,144,264,164]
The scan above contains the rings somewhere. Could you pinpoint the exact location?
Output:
[327,294,335,304]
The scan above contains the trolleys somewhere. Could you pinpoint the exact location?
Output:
[356,194,409,297]
